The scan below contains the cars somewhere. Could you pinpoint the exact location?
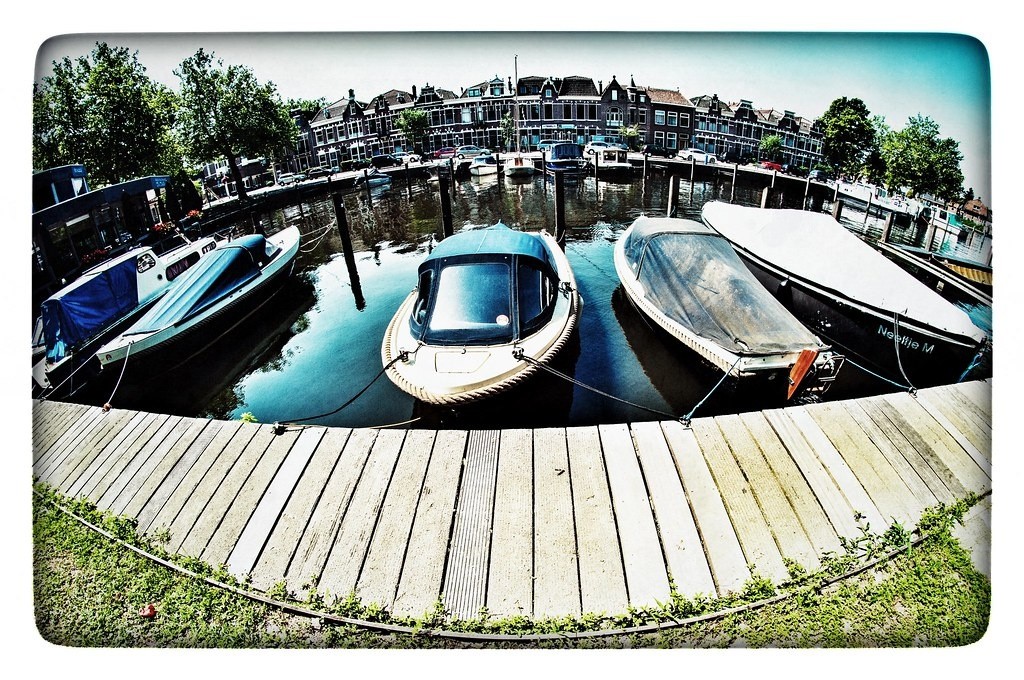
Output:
[351,159,372,171]
[307,167,335,180]
[720,150,847,185]
[454,145,492,161]
[435,146,456,159]
[278,172,307,187]
[585,141,620,156]
[425,150,440,161]
[678,148,717,164]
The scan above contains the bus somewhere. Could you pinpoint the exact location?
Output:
[536,138,574,152]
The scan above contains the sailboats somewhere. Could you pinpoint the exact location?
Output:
[502,54,535,177]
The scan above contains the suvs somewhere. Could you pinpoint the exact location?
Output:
[641,144,676,159]
[371,154,403,170]
[391,152,421,164]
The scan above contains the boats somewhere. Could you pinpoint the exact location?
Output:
[469,153,497,176]
[588,145,633,169]
[543,142,589,171]
[32,221,235,375]
[698,198,989,353]
[381,217,584,408]
[613,211,836,381]
[96,222,300,369]
[877,238,992,309]
